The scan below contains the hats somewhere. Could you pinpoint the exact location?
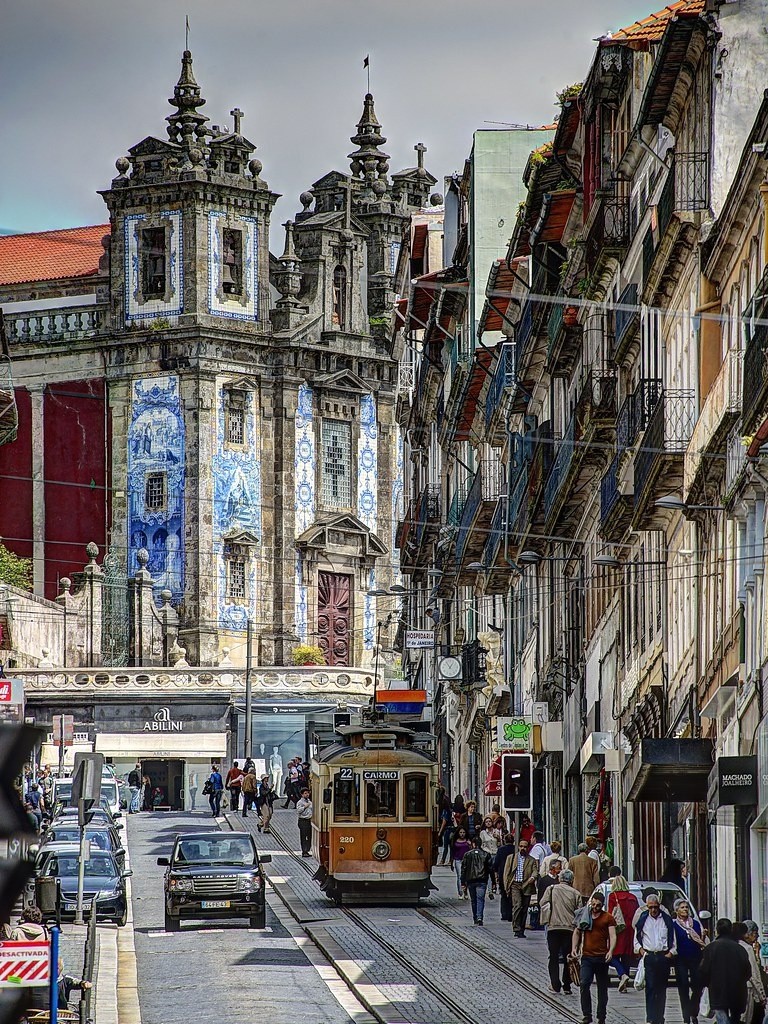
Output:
[558,869,574,883]
[261,774,269,780]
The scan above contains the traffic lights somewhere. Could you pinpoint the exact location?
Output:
[78,796,95,828]
[498,752,534,813]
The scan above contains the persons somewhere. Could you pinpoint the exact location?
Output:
[698,918,768,1024]
[503,838,538,938]
[257,773,274,834]
[461,836,496,926]
[572,892,617,1024]
[540,869,582,994]
[586,836,601,872]
[242,767,263,818]
[296,787,312,857]
[226,762,247,813]
[425,608,440,624]
[496,834,515,922]
[142,775,153,811]
[439,785,552,900]
[608,875,639,993]
[25,764,53,832]
[281,757,310,809]
[540,841,569,877]
[0,905,93,1024]
[243,757,255,773]
[210,766,224,818]
[608,866,621,878]
[632,887,708,1024]
[569,843,600,902]
[128,763,141,814]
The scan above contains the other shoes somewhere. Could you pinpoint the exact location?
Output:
[212,810,220,817]
[301,852,312,857]
[280,805,288,809]
[514,931,526,938]
[547,985,559,994]
[618,974,630,991]
[129,809,140,814]
[456,890,512,926]
[564,991,572,994]
[242,814,249,817]
[598,1019,605,1024]
[256,823,270,832]
[582,1017,593,1024]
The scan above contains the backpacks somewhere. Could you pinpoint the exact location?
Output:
[592,850,609,883]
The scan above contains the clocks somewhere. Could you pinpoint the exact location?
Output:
[439,656,462,678]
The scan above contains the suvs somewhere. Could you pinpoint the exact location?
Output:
[154,831,273,926]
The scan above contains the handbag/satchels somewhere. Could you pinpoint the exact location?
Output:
[293,781,301,787]
[612,907,626,934]
[220,793,228,808]
[699,987,715,1019]
[540,902,552,925]
[569,961,581,986]
[634,952,646,991]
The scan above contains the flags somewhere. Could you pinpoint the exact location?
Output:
[363,57,368,69]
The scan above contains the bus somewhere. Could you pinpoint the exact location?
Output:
[307,616,444,903]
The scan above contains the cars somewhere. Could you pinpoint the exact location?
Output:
[591,878,712,954]
[33,849,133,927]
[25,767,126,879]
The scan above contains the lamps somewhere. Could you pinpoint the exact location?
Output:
[518,551,585,563]
[427,569,477,578]
[366,585,432,595]
[591,555,666,567]
[654,496,728,511]
[543,659,580,695]
[465,562,517,571]
